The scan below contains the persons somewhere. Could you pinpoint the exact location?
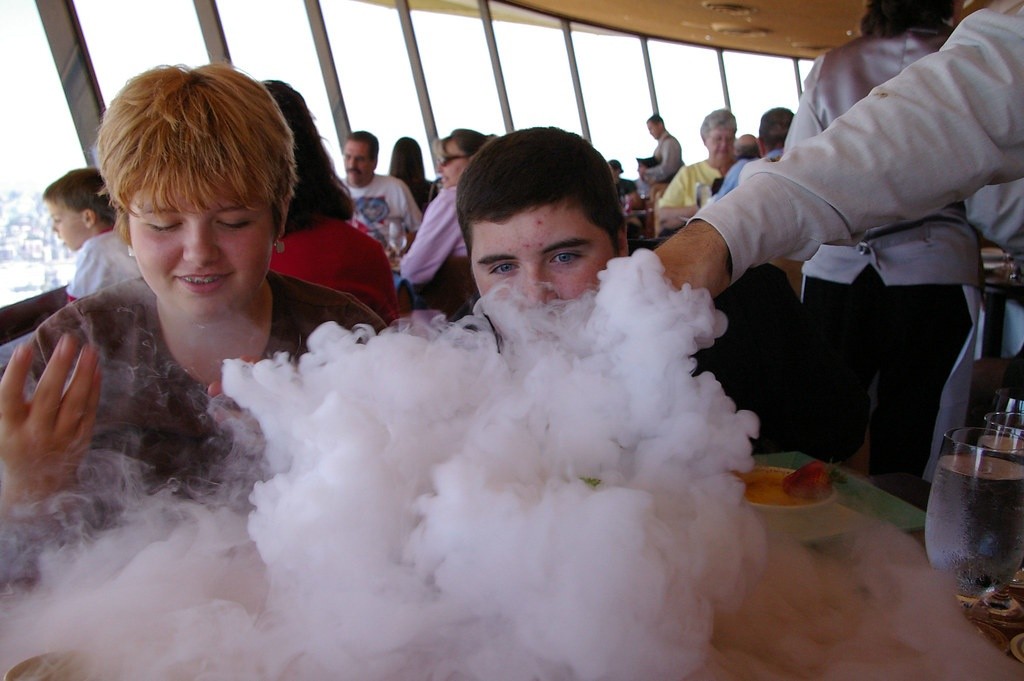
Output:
[659,3,1023,297]
[2,61,388,669]
[5,5,1023,493]
[454,127,631,507]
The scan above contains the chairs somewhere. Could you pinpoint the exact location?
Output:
[693,261,871,463]
[423,256,475,318]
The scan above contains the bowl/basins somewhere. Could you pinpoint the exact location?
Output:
[741,465,835,516]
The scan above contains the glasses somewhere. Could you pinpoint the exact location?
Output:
[440,154,466,166]
[709,134,735,145]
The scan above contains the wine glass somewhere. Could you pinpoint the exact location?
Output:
[922,389,1024,657]
[389,222,407,257]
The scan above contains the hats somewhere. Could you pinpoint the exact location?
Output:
[609,160,623,173]
